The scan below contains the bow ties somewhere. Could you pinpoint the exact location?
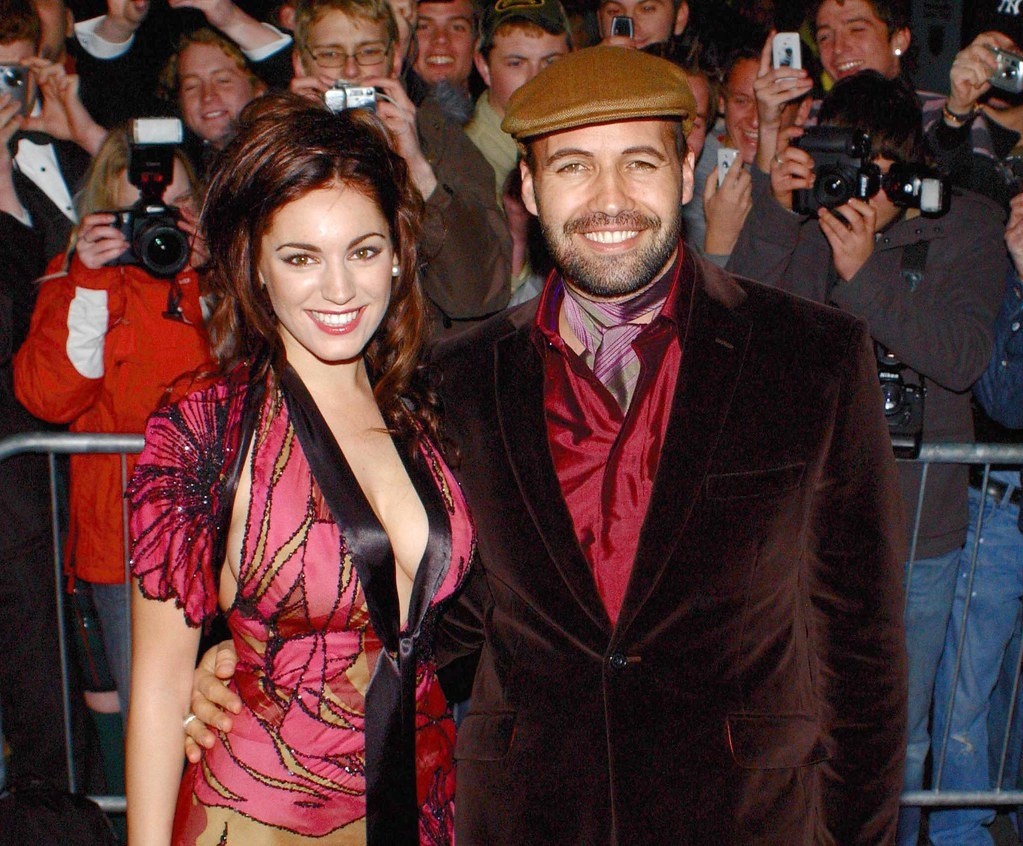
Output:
[7,130,54,159]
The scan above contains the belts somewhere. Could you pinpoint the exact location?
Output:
[968,470,1023,505]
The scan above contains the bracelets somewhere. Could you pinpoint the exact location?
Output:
[943,102,969,122]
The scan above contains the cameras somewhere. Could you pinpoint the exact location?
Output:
[986,49,1023,94]
[878,357,924,461]
[322,78,376,112]
[92,116,192,280]
[789,126,944,223]
[0,62,29,117]
[717,146,739,188]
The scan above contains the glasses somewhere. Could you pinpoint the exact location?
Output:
[303,39,391,67]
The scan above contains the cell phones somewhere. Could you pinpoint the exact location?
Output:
[772,31,802,83]
[610,15,634,40]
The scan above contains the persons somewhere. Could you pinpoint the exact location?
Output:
[122,95,476,846]
[184,44,913,846]
[0,0,1023,846]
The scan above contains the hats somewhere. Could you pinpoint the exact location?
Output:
[501,44,698,156]
[960,0,1023,51]
[479,0,567,39]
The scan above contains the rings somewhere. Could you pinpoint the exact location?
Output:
[83,236,94,243]
[183,715,195,728]
[775,154,783,164]
